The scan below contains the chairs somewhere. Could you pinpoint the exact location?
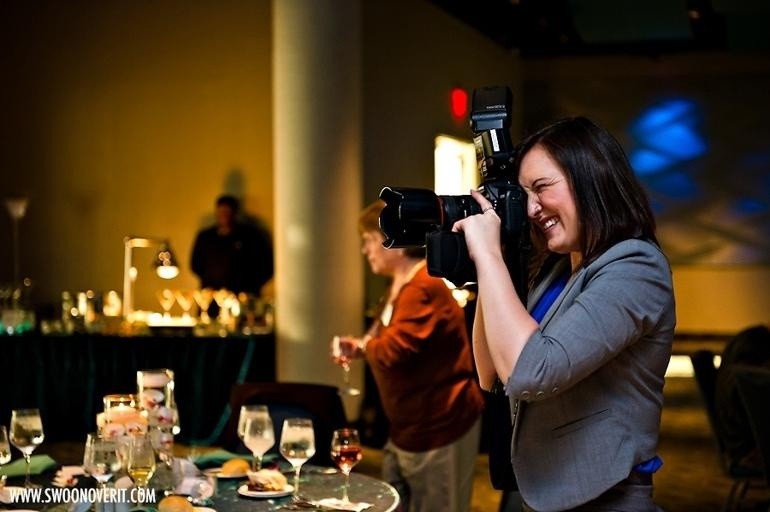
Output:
[688,322,770,512]
[230,380,349,468]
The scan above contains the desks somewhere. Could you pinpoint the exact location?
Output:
[1,315,275,441]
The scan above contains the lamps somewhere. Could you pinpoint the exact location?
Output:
[122,235,180,328]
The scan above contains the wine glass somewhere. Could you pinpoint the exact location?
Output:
[332,334,361,398]
[0,426,11,491]
[9,409,45,492]
[331,428,363,508]
[238,405,269,440]
[84,433,156,511]
[157,289,233,328]
[279,418,317,497]
[243,417,275,473]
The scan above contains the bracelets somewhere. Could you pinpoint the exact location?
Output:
[359,340,366,357]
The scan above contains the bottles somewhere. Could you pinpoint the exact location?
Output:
[57,289,97,326]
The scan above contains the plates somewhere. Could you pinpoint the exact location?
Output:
[204,467,253,480]
[236,481,295,500]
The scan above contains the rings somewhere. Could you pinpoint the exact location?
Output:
[483,207,493,214]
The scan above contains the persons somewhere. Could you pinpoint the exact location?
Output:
[187,192,275,326]
[328,199,485,512]
[449,112,676,512]
